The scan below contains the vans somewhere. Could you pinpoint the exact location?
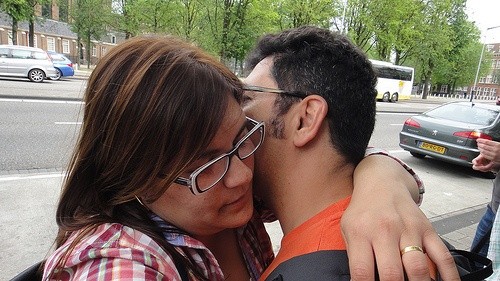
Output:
[0,44,56,83]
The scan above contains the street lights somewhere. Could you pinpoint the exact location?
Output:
[470,25,500,102]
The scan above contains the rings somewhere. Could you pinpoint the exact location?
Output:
[401,245,423,254]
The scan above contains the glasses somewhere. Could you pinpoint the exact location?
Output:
[238,84,307,115]
[152,113,265,197]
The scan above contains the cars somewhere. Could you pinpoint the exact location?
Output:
[46,50,74,81]
[399,100,500,178]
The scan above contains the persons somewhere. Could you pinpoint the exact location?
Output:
[239,25,468,281]
[470,133,500,281]
[38,31,460,281]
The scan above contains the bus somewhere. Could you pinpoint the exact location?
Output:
[366,57,414,103]
